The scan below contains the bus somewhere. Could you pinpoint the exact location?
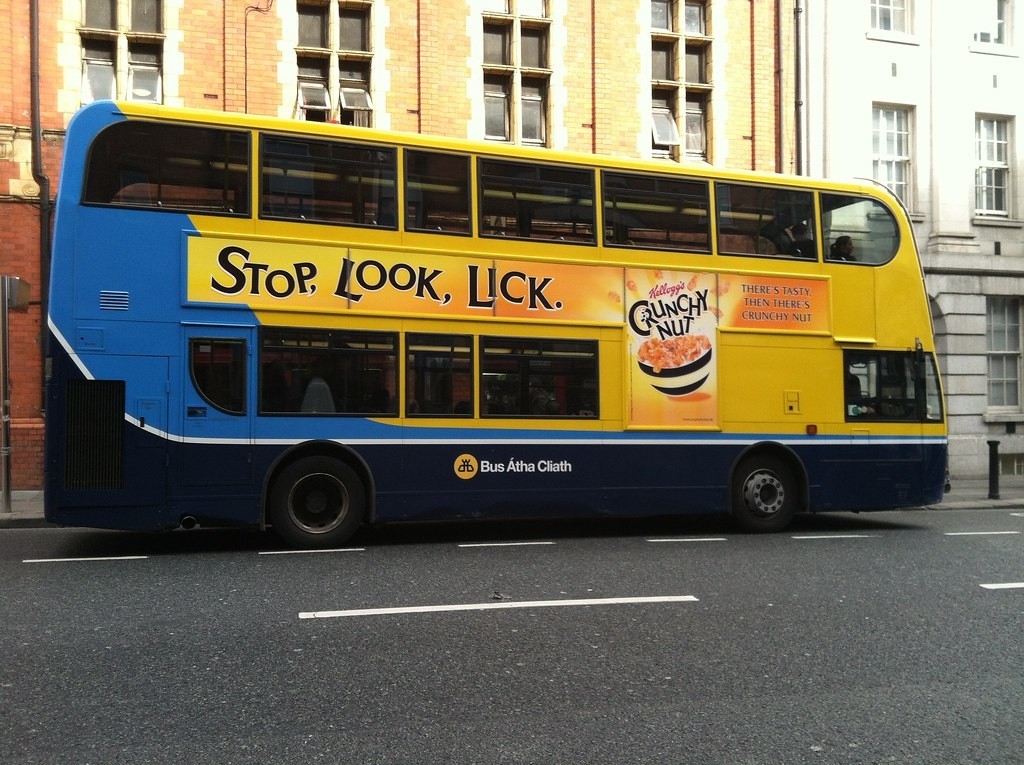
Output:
[39,99,954,552]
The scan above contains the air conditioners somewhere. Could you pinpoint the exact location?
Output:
[973,31,994,43]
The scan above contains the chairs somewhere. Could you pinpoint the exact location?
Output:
[155,200,847,262]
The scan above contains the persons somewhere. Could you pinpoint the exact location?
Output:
[377,196,394,226]
[788,224,816,258]
[829,236,855,261]
